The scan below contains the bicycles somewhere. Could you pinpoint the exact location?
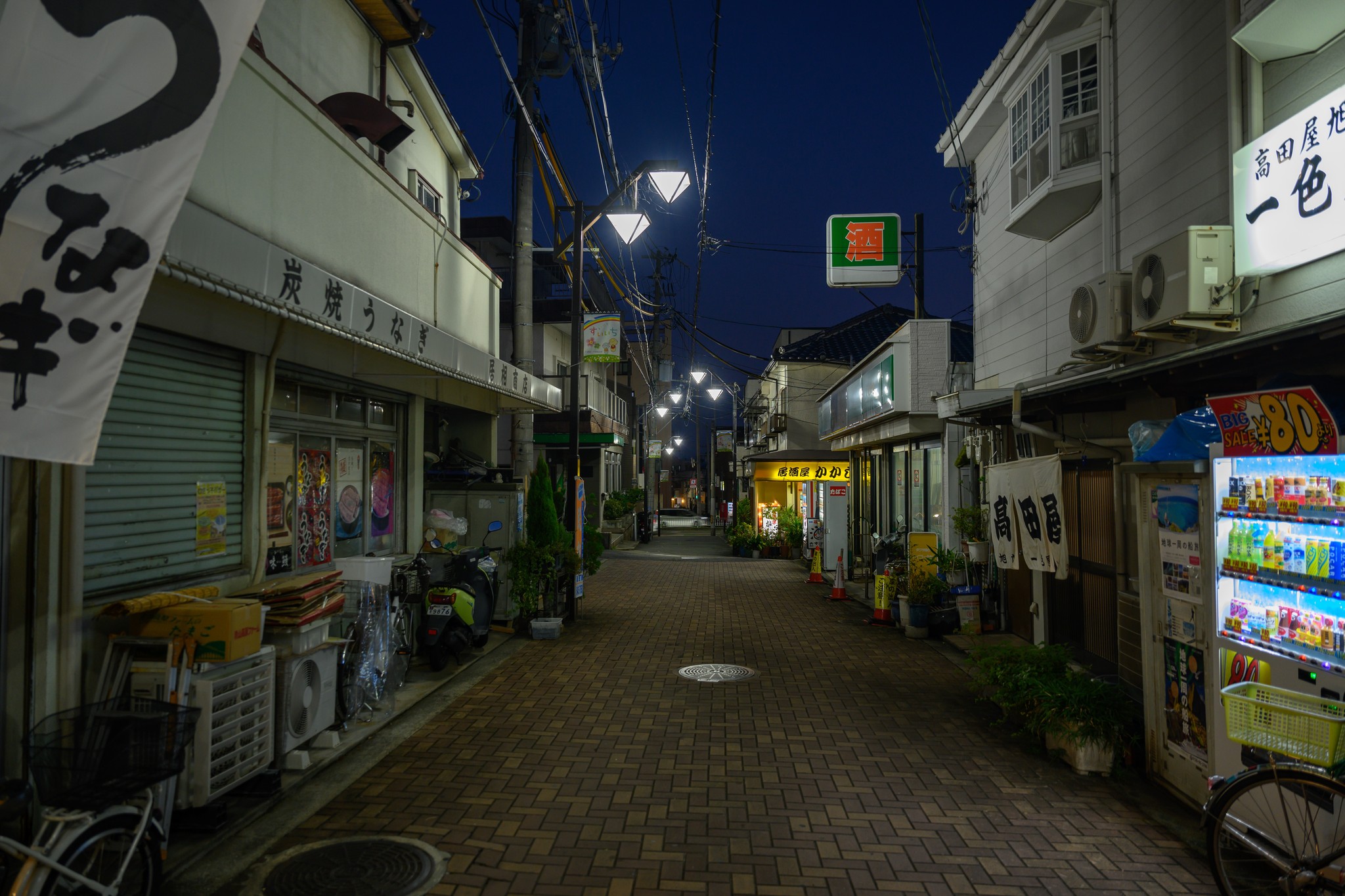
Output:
[0,693,202,895]
[1201,681,1345,895]
[336,526,437,735]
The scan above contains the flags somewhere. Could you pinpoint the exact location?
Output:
[0,0,269,465]
[988,453,1069,579]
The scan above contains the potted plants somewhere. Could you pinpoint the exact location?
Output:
[502,447,605,616]
[953,622,1144,778]
[602,483,647,529]
[725,493,807,560]
[882,474,991,638]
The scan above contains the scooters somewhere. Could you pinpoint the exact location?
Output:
[872,515,908,599]
[632,507,653,544]
[415,520,504,671]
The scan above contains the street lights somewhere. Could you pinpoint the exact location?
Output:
[551,160,690,638]
[635,368,743,537]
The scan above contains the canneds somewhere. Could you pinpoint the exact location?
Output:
[1227,475,1345,654]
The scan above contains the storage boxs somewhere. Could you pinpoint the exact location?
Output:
[1220,680,1345,769]
[22,690,203,815]
[529,617,563,639]
[130,556,395,663]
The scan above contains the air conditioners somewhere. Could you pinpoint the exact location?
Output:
[1068,270,1153,361]
[1130,225,1241,344]
[129,642,338,810]
[757,428,765,444]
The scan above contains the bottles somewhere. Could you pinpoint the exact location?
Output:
[1218,465,1345,665]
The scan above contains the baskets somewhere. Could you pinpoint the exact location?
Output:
[1219,680,1345,769]
[485,571,498,593]
[20,694,205,811]
[390,564,431,604]
[870,535,904,554]
[332,578,389,620]
[416,552,468,588]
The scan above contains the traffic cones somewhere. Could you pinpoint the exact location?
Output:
[863,569,899,626]
[823,556,854,602]
[804,545,827,585]
